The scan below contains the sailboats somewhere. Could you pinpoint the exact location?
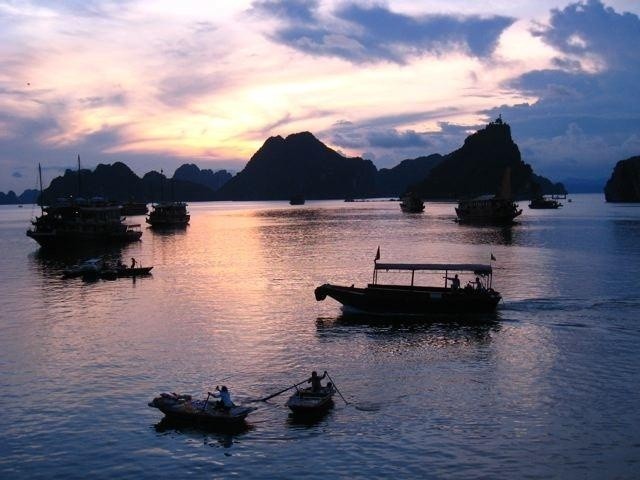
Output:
[25,153,192,252]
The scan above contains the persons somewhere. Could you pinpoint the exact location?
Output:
[117,260,122,267]
[209,386,233,415]
[131,257,137,269]
[184,395,192,413]
[447,274,460,290]
[469,277,481,290]
[514,204,519,213]
[308,371,327,393]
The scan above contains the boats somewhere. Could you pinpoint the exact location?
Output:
[147,391,260,421]
[287,191,307,206]
[283,382,336,417]
[314,261,505,320]
[400,190,426,214]
[450,181,575,228]
[58,253,154,282]
